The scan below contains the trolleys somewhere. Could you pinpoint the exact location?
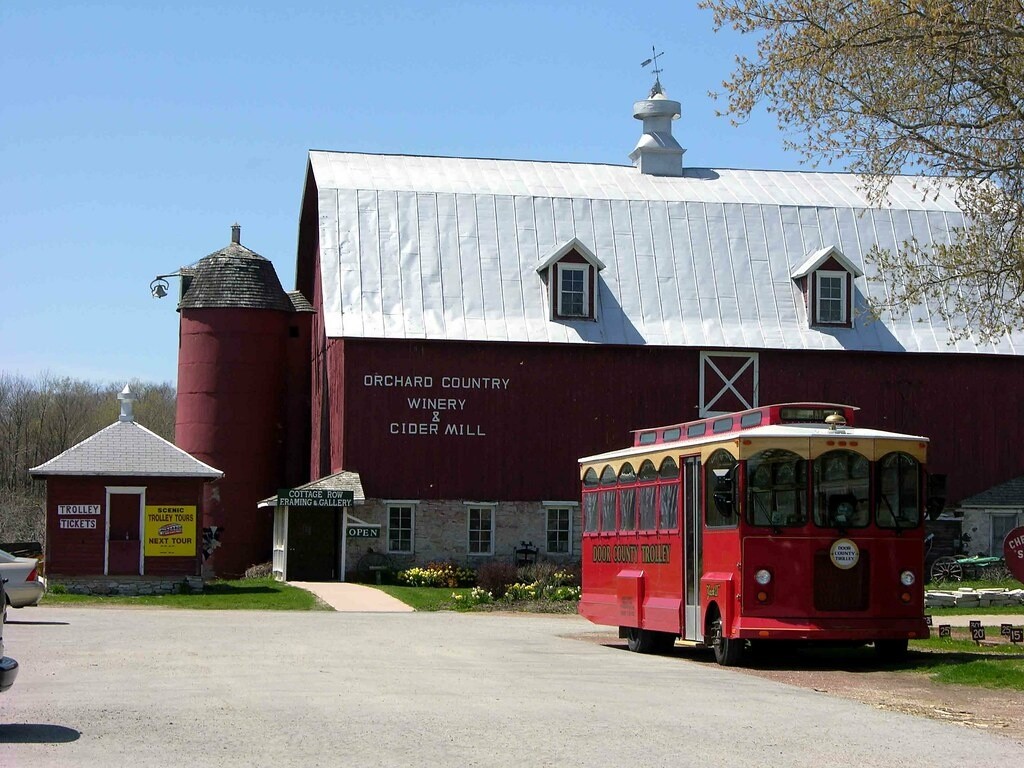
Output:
[929,554,1006,588]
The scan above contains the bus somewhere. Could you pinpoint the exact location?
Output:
[576,402,930,670]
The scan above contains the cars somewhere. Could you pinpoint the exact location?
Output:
[0,550,45,608]
[0,576,20,692]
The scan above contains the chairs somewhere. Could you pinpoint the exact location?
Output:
[829,494,854,529]
